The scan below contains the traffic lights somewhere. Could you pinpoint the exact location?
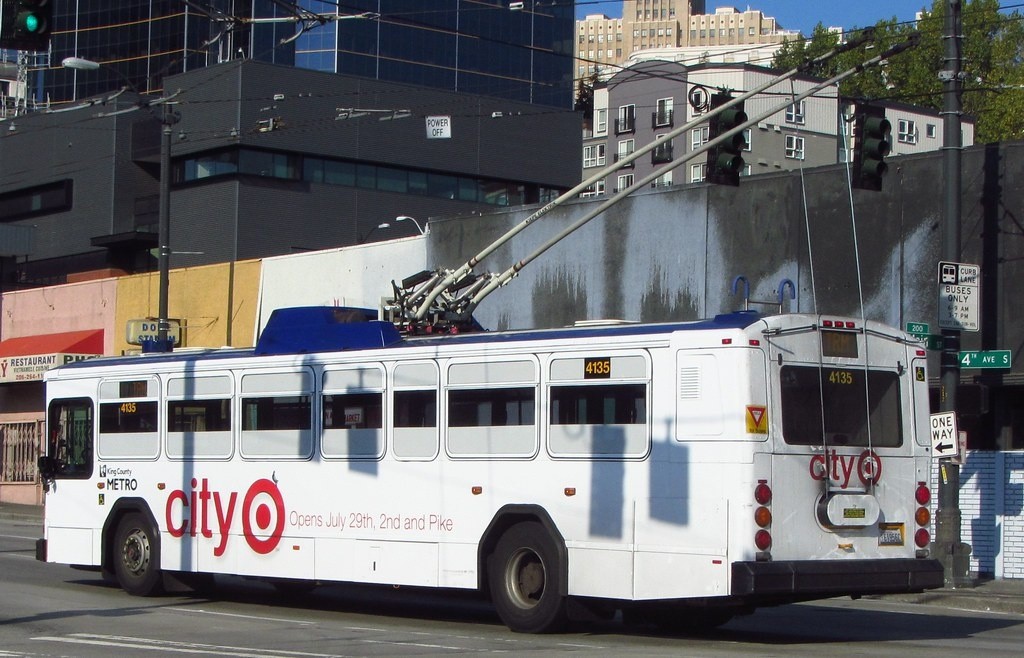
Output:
[11,0,48,38]
[706,94,747,187]
[852,103,892,193]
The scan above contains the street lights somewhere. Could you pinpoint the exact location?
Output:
[62,55,171,355]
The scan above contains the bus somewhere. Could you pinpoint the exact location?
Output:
[37,27,947,636]
